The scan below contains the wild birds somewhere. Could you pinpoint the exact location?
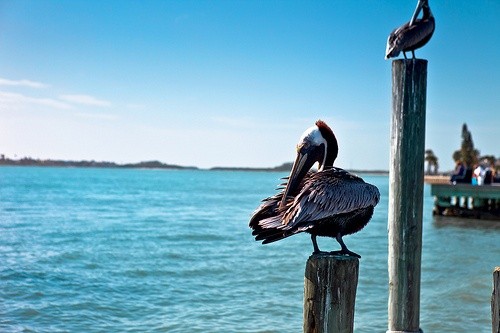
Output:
[250,120,380,258]
[384,0,436,59]
